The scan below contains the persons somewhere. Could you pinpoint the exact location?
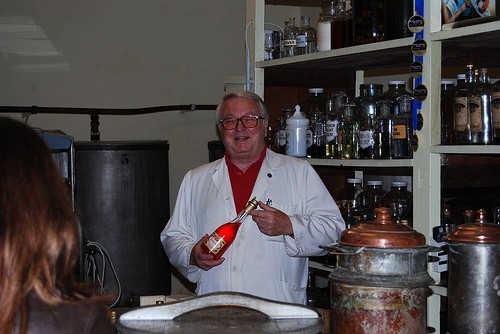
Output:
[160,90,346,305]
[0,116,119,334]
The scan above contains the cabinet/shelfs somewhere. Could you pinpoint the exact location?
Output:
[244,0,500,334]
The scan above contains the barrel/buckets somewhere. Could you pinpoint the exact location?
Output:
[442,209,500,334]
[318,207,441,334]
[72,139,172,306]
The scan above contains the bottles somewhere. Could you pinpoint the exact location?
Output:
[107,308,119,334]
[272,16,316,60]
[200,195,259,262]
[324,0,346,17]
[344,179,412,230]
[276,81,413,160]
[441,64,500,145]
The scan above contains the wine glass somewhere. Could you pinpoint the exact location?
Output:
[264,33,273,61]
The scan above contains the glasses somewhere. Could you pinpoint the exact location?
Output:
[219,114,266,130]
